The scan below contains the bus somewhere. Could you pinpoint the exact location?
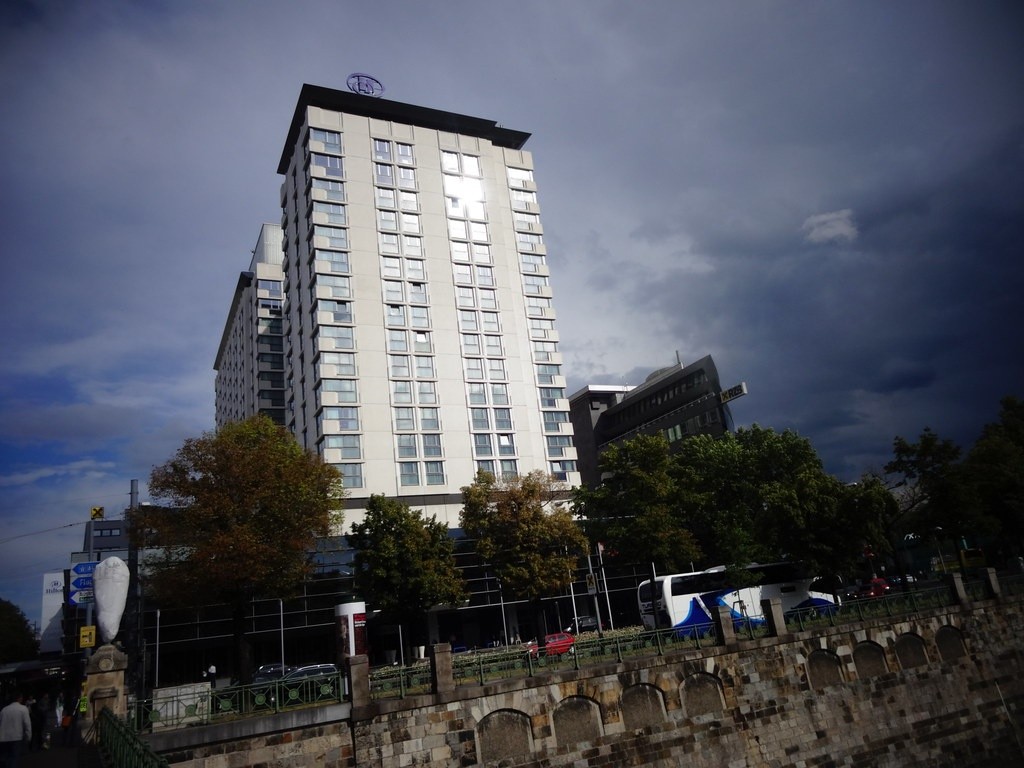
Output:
[637,560,843,641]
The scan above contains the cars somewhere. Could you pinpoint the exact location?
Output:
[852,578,891,600]
[522,633,574,659]
[572,616,597,633]
[212,663,340,711]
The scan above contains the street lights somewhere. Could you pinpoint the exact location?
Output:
[932,527,947,576]
[579,502,606,656]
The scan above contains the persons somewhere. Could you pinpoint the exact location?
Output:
[59,678,82,749]
[0,686,33,768]
[24,692,47,751]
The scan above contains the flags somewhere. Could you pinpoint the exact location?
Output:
[599,538,621,557]
